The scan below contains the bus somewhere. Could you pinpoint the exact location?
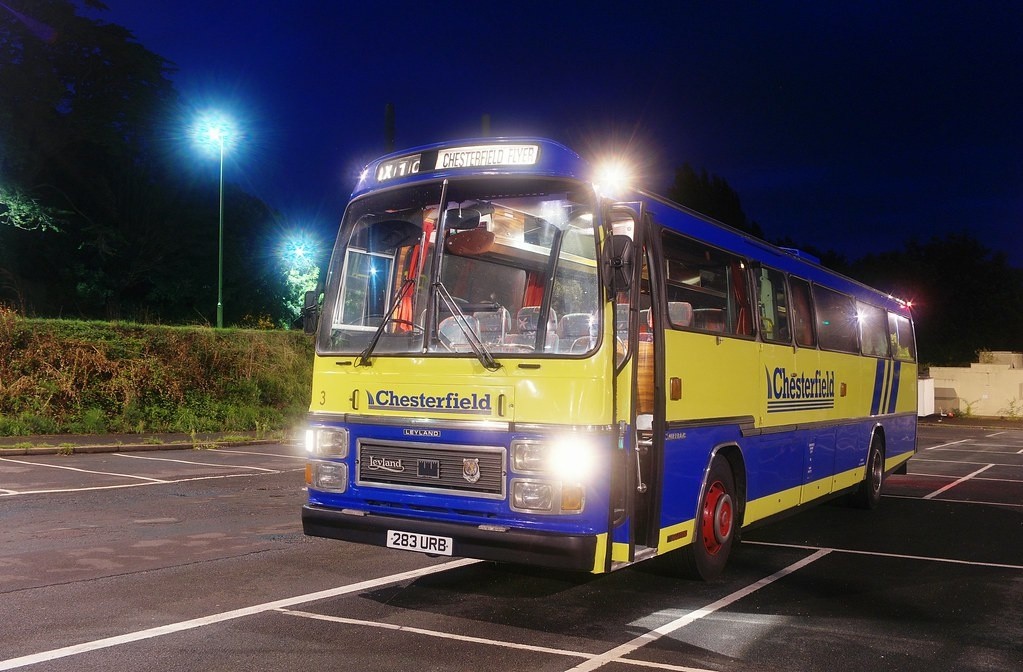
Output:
[301,133,922,583]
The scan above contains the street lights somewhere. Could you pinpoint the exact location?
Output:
[203,123,227,332]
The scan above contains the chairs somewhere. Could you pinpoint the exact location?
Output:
[472,305,512,354]
[594,303,629,341]
[513,306,560,354]
[557,312,595,354]
[436,314,481,351]
[646,302,693,344]
